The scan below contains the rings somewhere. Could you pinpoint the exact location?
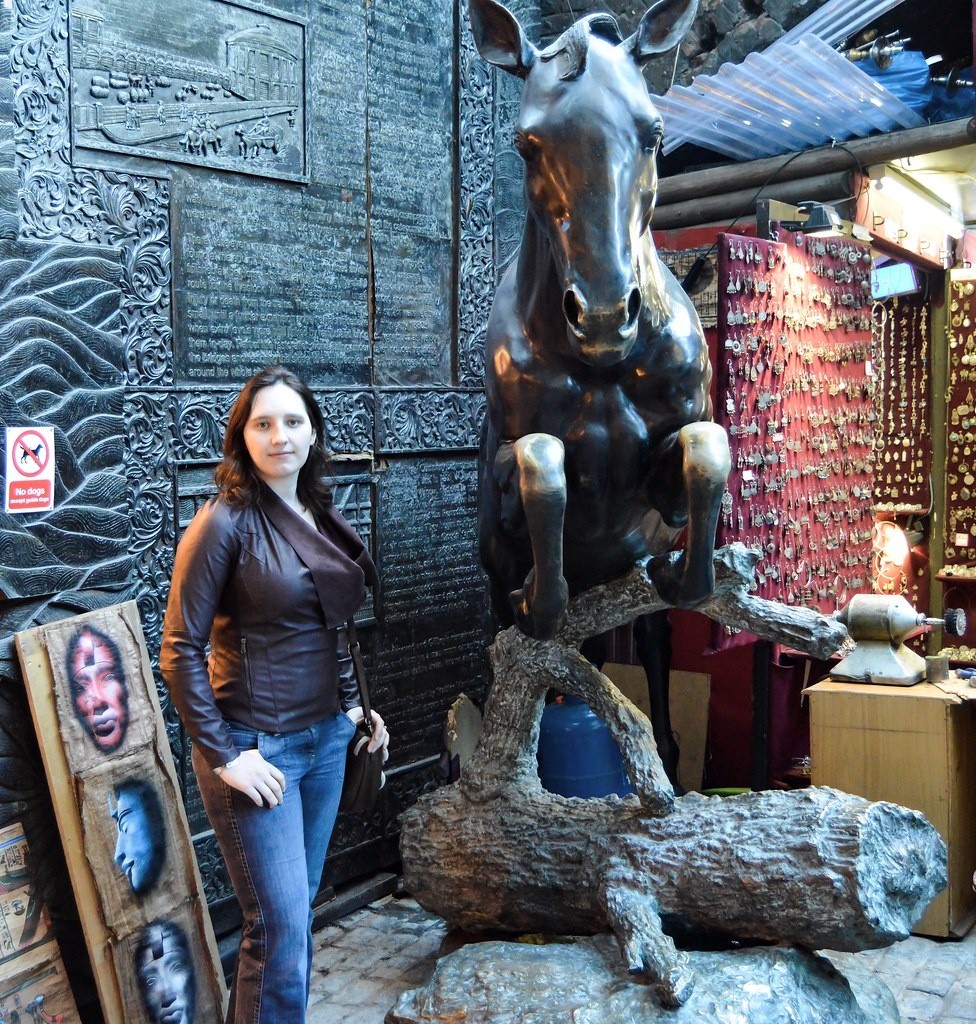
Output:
[384,726,387,729]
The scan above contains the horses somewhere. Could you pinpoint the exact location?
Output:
[476,1,735,643]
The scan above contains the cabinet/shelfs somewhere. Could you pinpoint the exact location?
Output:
[801,670,976,938]
[934,571,976,668]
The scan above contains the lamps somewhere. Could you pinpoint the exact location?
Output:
[867,164,965,240]
[780,205,845,237]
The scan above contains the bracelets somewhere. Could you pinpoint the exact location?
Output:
[213,759,238,775]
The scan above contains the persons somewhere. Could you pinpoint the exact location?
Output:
[158,363,389,1024]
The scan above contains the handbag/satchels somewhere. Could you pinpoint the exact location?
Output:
[339,729,383,815]
